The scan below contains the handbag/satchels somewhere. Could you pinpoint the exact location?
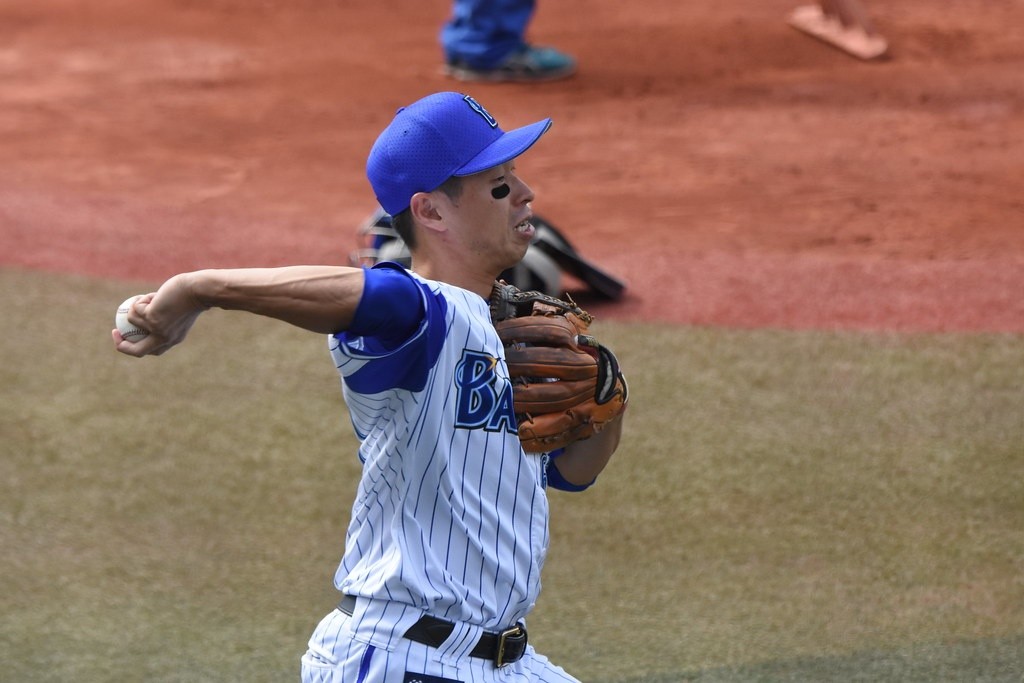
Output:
[372,212,629,304]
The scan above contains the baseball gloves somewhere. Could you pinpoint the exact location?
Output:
[490,276,631,458]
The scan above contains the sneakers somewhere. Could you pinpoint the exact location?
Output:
[443,42,577,82]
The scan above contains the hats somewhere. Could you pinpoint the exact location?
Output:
[366,92,552,217]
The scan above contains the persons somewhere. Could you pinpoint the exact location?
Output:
[111,92,628,683]
[439,0,578,83]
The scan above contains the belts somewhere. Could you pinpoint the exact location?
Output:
[337,594,528,668]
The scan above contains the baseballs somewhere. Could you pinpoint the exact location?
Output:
[114,293,151,344]
